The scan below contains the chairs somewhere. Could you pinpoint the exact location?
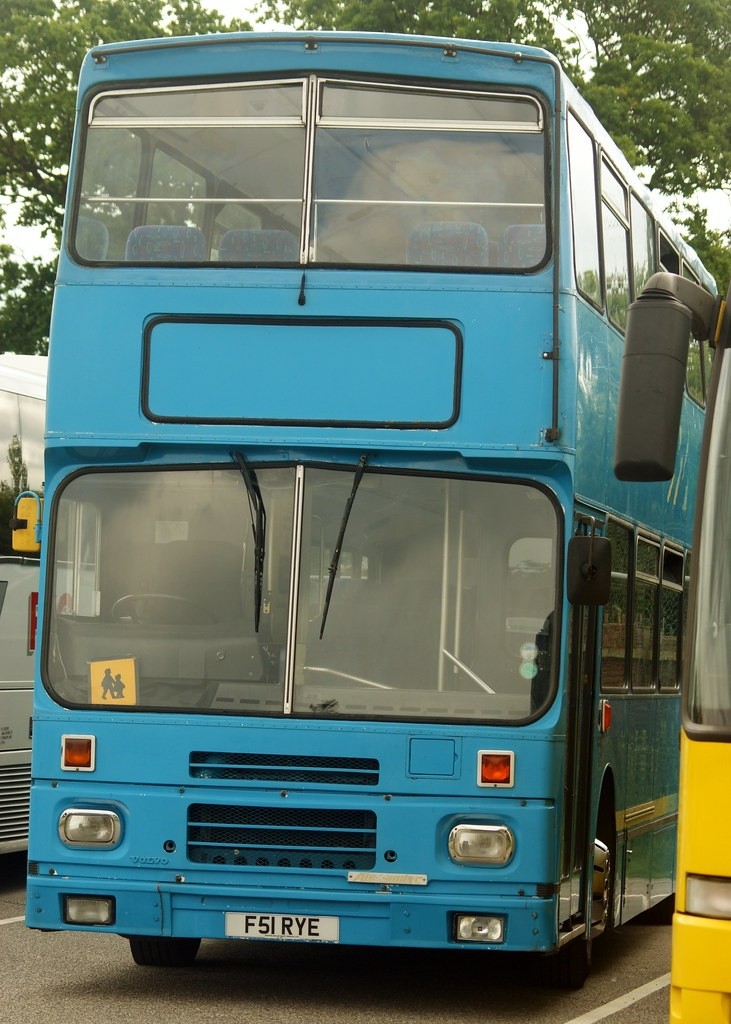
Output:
[404,220,489,268]
[74,215,110,262]
[124,224,208,263]
[216,228,299,263]
[497,224,546,269]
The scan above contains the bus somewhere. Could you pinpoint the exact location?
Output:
[20,32,713,1003]
[613,268,731,1024]
[0,352,47,862]
